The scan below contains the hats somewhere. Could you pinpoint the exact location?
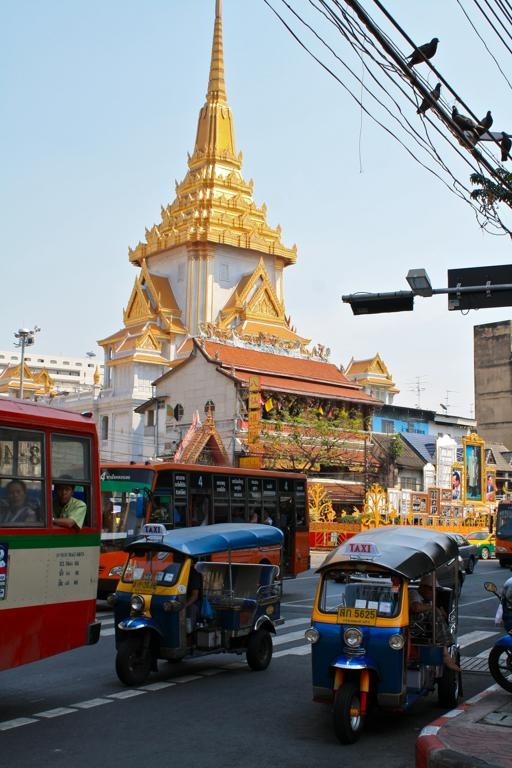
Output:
[421,575,442,587]
[409,590,424,602]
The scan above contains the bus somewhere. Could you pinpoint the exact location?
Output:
[0,395,101,673]
[495,497,512,568]
[96,461,311,600]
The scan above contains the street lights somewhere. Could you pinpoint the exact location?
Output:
[12,322,42,402]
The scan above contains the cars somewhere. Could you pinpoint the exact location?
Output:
[463,530,496,560]
[444,531,478,575]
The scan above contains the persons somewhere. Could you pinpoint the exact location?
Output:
[169,502,182,524]
[371,575,400,614]
[232,507,304,528]
[0,480,37,523]
[196,492,209,526]
[408,575,463,672]
[499,517,511,531]
[102,502,113,532]
[161,550,203,654]
[52,476,87,529]
[487,475,494,501]
[468,447,478,497]
[452,472,462,500]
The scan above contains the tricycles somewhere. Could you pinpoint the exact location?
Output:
[303,524,469,744]
[107,522,288,685]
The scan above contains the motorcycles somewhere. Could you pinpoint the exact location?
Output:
[482,577,512,692]
